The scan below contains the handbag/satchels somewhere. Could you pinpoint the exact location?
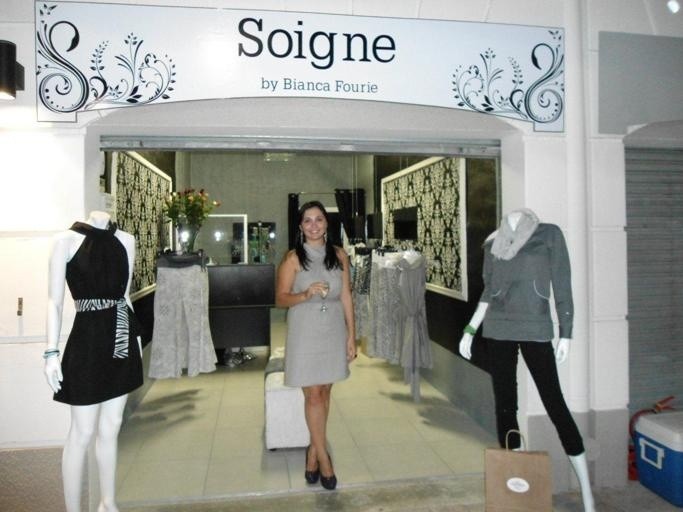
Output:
[484,429,551,511]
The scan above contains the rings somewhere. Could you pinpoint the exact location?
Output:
[353,354,357,357]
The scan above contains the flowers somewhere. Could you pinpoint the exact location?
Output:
[164,186,221,224]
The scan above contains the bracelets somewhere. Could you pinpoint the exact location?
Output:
[44,350,59,357]
[463,324,478,336]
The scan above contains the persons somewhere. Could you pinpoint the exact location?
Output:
[42,212,144,512]
[273,200,357,493]
[458,209,596,512]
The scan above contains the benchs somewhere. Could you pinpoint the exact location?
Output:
[262,345,311,451]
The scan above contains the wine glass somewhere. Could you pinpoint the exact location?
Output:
[318,282,330,312]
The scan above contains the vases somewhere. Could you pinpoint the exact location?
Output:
[177,227,198,254]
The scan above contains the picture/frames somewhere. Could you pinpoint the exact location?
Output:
[111,150,173,305]
[381,151,469,304]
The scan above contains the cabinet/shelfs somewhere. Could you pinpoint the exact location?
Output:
[149,251,212,378]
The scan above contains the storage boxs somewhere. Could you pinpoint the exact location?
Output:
[633,411,682,508]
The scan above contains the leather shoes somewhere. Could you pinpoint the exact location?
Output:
[317,454,337,489]
[305,444,319,483]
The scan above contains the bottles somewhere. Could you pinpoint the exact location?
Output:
[248,233,258,265]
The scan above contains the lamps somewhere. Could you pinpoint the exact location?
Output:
[0,40,25,101]
